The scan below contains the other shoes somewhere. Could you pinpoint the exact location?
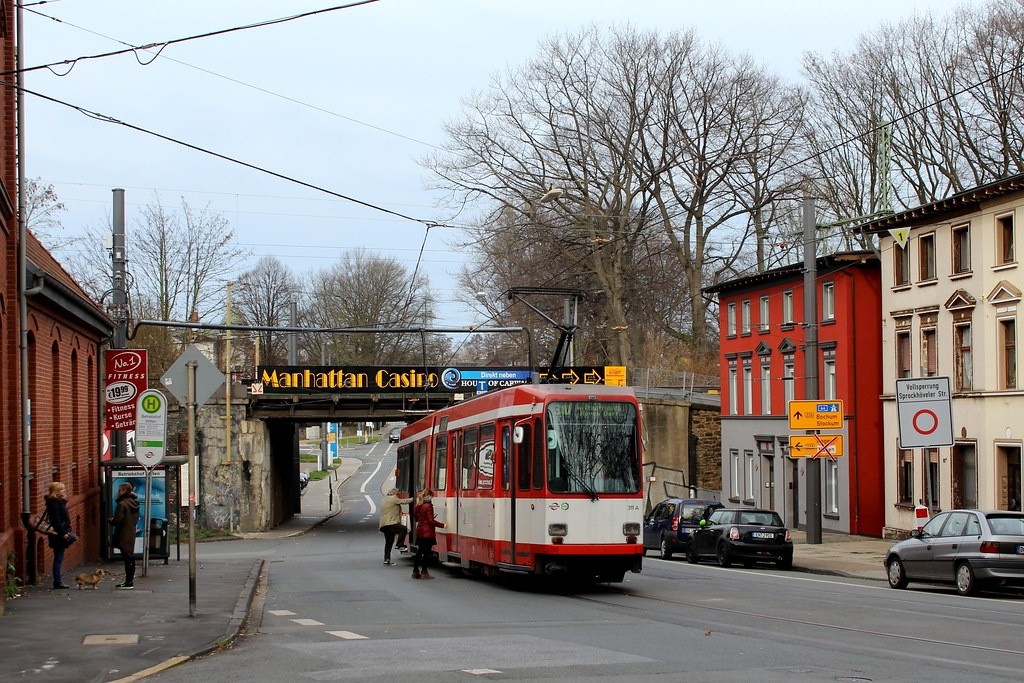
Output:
[412,569,422,578]
[421,570,435,580]
[115,582,134,589]
[384,561,396,566]
[395,546,407,551]
[54,583,69,589]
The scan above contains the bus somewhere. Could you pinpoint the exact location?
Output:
[394,286,649,584]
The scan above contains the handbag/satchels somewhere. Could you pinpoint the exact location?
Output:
[20,508,58,535]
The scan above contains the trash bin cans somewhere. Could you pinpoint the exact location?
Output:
[149,517,171,565]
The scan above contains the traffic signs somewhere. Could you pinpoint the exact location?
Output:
[789,401,844,430]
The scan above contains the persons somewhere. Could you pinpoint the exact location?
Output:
[43,481,78,589]
[379,488,414,567]
[108,483,140,590]
[413,488,447,581]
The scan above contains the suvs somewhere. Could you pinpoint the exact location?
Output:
[644,498,725,560]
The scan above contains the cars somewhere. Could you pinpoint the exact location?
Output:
[883,509,1024,596]
[389,429,401,443]
[687,508,793,570]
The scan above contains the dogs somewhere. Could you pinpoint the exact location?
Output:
[74,569,106,590]
